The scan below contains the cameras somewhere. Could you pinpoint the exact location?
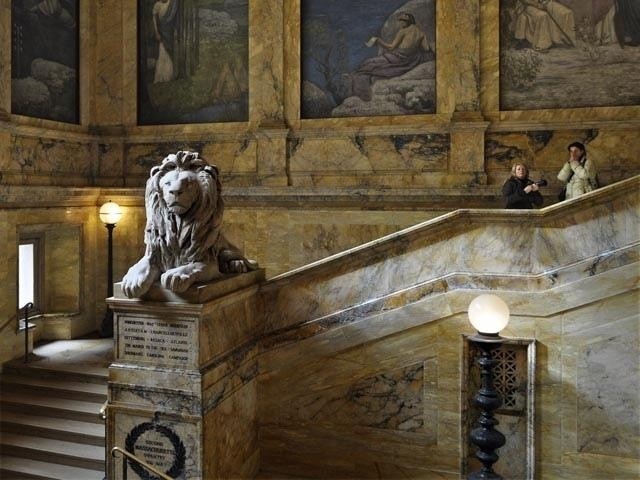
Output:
[526,179,547,187]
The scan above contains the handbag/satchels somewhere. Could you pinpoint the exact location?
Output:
[558,186,566,200]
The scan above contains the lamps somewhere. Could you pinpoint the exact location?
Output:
[99,201,121,337]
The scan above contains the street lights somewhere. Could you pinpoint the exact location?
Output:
[92,199,129,338]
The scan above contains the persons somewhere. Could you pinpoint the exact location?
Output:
[510,1,576,54]
[503,163,543,209]
[595,0,640,46]
[342,12,430,100]
[557,142,598,200]
[152,0,182,82]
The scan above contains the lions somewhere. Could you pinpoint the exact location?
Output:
[120,151,258,298]
